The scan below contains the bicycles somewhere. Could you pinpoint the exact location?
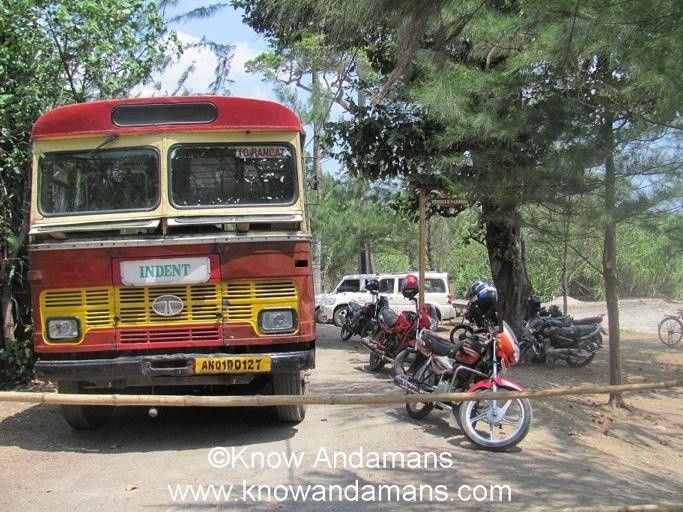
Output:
[658,309,683,347]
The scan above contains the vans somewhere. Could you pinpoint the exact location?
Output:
[315,271,456,328]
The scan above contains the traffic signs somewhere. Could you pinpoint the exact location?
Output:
[430,199,470,207]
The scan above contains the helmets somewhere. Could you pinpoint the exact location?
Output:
[465,281,498,309]
[527,295,542,309]
[401,275,418,297]
[366,279,380,291]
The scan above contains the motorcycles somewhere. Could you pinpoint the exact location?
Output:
[360,290,438,371]
[338,288,388,340]
[394,305,533,452]
[450,281,608,369]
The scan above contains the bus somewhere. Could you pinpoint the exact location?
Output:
[27,95,316,431]
